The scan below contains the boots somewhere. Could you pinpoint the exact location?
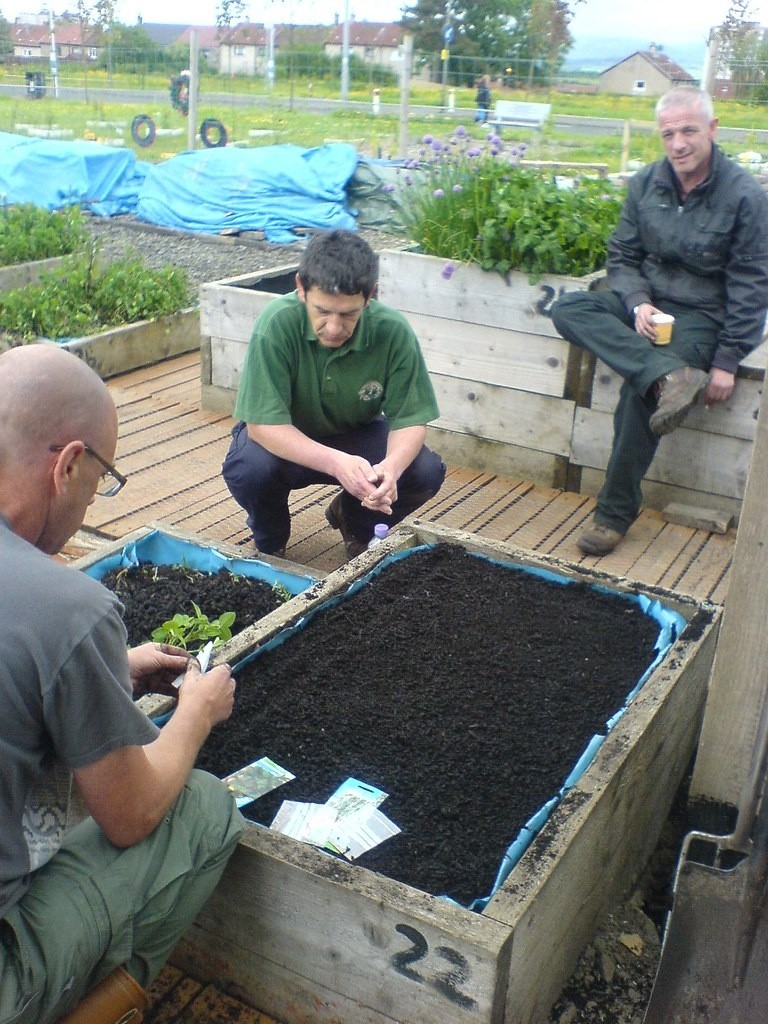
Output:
[54,965,151,1023]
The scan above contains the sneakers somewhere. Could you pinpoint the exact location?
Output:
[648,365,712,435]
[325,492,369,558]
[576,521,626,556]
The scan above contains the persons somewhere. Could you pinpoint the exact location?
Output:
[473,77,493,121]
[0,341,245,1024]
[222,228,447,561]
[550,85,768,557]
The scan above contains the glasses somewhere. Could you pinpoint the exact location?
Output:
[47,440,129,501]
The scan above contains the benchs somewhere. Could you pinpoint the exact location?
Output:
[486,99,554,142]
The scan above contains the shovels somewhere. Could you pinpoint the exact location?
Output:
[639,693,768,1024]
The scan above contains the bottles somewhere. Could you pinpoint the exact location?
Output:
[368,524,389,549]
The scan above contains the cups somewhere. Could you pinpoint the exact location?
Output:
[650,313,675,345]
